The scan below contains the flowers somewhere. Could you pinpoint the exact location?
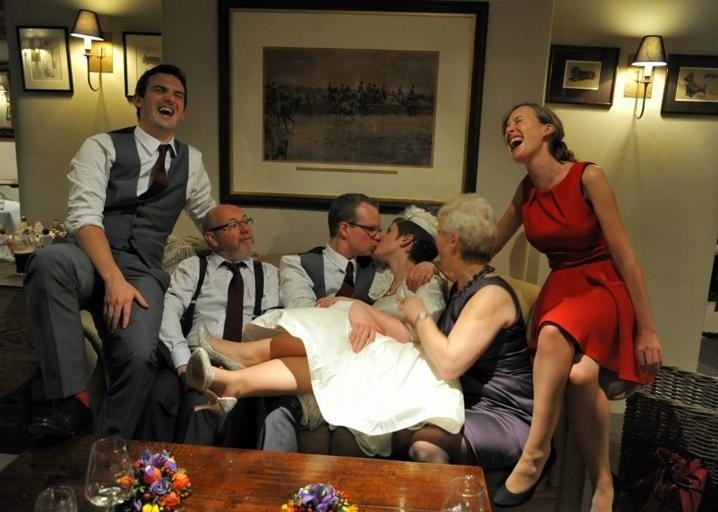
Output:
[277,477,359,511]
[107,444,196,511]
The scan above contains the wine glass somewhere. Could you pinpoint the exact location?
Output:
[83,439,134,511]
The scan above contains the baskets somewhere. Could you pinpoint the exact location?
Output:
[617,361,718,492]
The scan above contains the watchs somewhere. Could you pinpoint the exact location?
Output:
[412,310,432,329]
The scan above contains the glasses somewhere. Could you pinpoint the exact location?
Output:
[347,220,381,238]
[205,217,254,233]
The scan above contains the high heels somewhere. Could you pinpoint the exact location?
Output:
[184,348,240,417]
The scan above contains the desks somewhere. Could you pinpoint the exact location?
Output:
[0,417,494,511]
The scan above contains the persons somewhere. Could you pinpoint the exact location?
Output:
[253,191,445,458]
[22,62,218,473]
[380,190,558,471]
[184,201,449,422]
[481,101,665,510]
[133,200,281,452]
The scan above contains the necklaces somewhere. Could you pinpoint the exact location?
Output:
[385,277,404,294]
[443,264,495,301]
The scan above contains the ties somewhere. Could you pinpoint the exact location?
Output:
[334,261,357,298]
[220,260,248,344]
[148,144,172,193]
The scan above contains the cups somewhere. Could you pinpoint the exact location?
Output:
[15,254,32,276]
[35,486,76,512]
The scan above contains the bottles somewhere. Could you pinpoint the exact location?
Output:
[0,213,63,251]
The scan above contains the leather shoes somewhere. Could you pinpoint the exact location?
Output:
[492,438,559,508]
[197,318,248,371]
[29,395,96,441]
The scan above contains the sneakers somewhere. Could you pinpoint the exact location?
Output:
[608,471,622,512]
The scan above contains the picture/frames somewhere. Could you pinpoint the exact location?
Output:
[122,30,164,100]
[544,42,622,108]
[658,51,718,117]
[14,24,76,99]
[0,60,19,140]
[214,0,490,222]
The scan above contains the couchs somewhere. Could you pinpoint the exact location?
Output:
[52,231,645,510]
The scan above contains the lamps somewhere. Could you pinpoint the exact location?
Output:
[621,32,669,121]
[69,6,116,94]
